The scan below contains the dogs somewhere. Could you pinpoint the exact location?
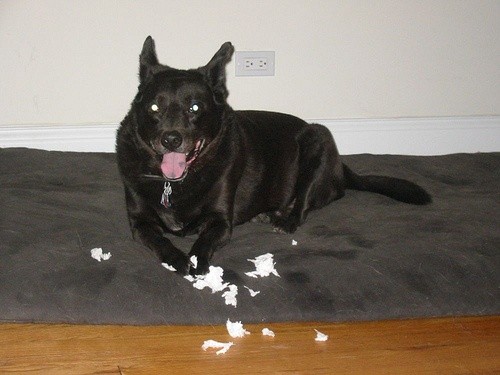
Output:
[115,35,433,277]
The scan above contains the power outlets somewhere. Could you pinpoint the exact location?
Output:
[235,51,276,77]
